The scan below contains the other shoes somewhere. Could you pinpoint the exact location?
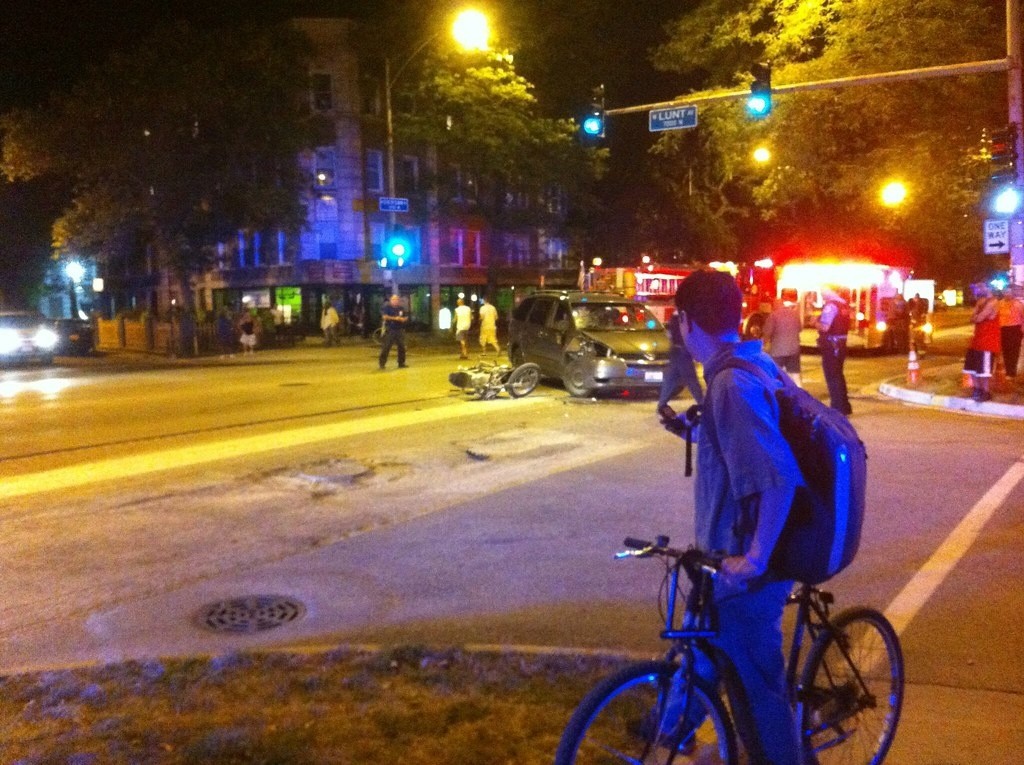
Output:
[839,406,852,413]
[976,391,991,401]
[398,363,408,368]
[380,364,386,368]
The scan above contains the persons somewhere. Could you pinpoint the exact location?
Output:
[762,297,805,387]
[742,292,774,353]
[911,293,927,328]
[884,289,910,355]
[602,308,620,325]
[480,294,500,356]
[379,294,408,369]
[320,300,340,346]
[807,282,854,414]
[962,277,1024,403]
[237,309,258,357]
[624,264,806,765]
[452,299,474,358]
[657,310,705,412]
[217,301,237,360]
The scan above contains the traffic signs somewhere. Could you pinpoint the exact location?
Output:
[983,218,1009,256]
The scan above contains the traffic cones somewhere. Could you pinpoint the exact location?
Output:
[907,339,920,387]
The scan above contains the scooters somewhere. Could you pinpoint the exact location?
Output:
[448,356,541,398]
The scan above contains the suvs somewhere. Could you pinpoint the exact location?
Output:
[506,288,684,398]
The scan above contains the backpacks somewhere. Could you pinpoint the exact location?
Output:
[707,354,868,583]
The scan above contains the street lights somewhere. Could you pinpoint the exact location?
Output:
[385,9,493,236]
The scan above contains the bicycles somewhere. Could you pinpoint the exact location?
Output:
[554,534,904,765]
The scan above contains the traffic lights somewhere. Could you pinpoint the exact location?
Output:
[584,82,608,140]
[990,121,1018,187]
[746,60,774,118]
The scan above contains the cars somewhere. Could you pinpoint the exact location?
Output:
[0,310,91,369]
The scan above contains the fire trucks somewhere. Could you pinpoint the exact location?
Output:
[774,260,937,353]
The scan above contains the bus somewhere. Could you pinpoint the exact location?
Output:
[587,267,693,322]
